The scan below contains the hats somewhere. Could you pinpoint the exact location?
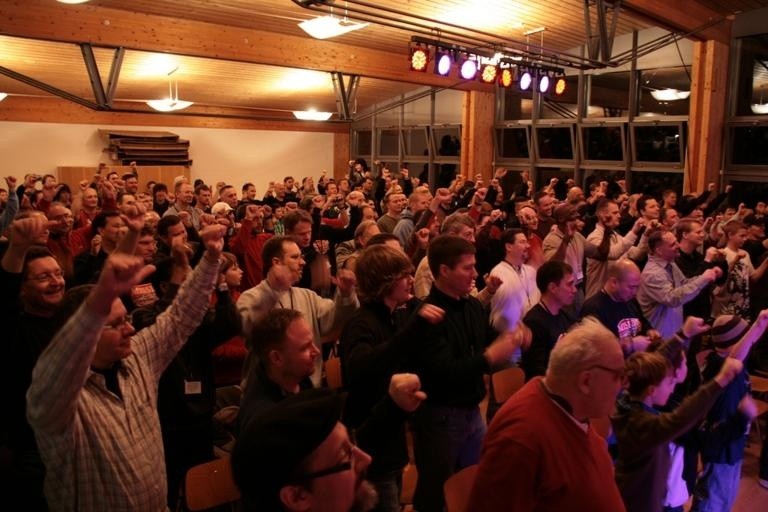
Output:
[211,200,235,216]
[712,311,753,353]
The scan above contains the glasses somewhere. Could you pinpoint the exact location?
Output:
[103,314,134,332]
[53,213,73,224]
[301,426,361,481]
[462,229,477,242]
[290,253,307,263]
[584,364,636,386]
[37,268,66,281]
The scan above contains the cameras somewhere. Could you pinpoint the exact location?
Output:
[334,196,343,202]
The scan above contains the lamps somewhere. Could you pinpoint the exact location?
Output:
[295,3,369,44]
[140,67,195,114]
[287,107,335,123]
[406,24,567,101]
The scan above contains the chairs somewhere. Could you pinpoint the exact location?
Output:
[47,208,767,512]
